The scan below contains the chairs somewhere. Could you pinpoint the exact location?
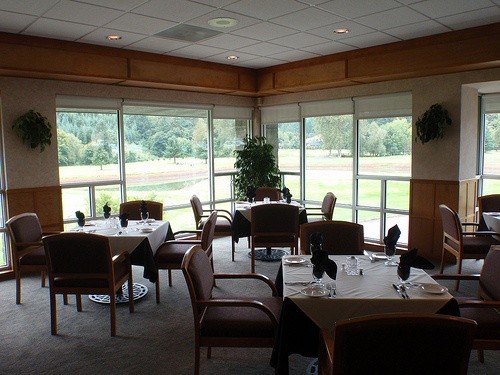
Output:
[4,187,500,375]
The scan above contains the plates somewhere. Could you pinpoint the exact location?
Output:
[301,287,327,297]
[141,229,154,233]
[148,219,155,223]
[285,257,306,263]
[422,284,444,294]
[372,253,387,259]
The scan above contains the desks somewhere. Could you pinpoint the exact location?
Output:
[60,220,175,314]
[230,201,309,261]
[269,255,462,375]
[483,212,500,241]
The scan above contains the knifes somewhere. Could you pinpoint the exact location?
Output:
[393,284,405,298]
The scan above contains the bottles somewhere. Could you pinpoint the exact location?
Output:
[110,217,119,229]
[264,197,270,204]
[346,256,357,276]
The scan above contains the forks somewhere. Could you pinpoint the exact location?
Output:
[332,283,336,296]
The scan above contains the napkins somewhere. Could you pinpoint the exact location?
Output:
[74,210,85,226]
[103,202,111,219]
[118,213,128,227]
[310,250,337,281]
[398,248,435,280]
[140,200,149,219]
[383,224,401,256]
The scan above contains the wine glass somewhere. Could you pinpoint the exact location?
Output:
[141,212,149,226]
